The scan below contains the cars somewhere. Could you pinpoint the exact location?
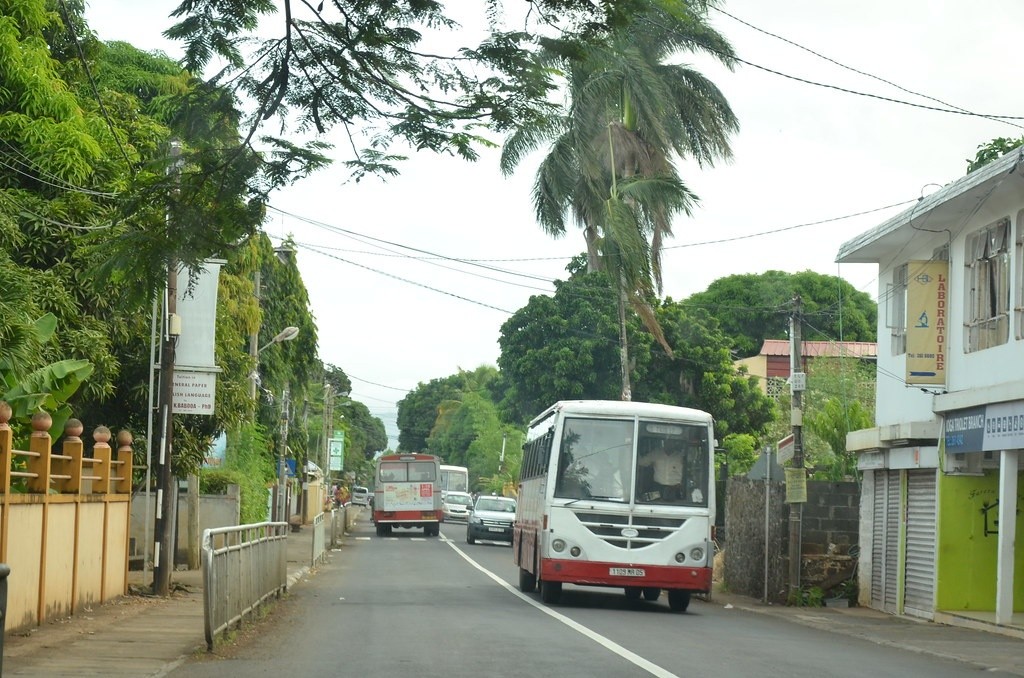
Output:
[441,490,475,524]
[464,495,517,547]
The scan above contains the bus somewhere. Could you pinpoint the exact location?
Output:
[440,464,470,502]
[369,452,443,537]
[512,398,717,613]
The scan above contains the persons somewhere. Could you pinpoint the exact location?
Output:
[331,483,349,509]
[638,435,697,500]
[562,444,623,500]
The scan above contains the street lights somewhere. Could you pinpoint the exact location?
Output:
[299,381,333,524]
[242,324,301,457]
[322,390,349,480]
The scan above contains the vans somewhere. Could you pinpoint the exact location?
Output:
[351,486,369,506]
